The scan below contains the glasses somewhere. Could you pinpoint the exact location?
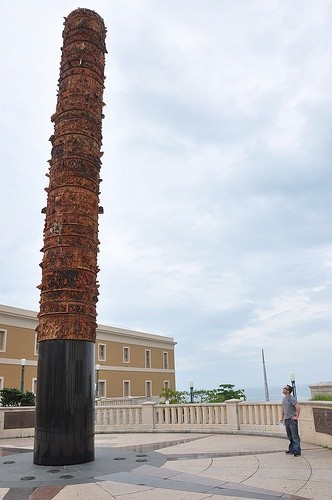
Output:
[283,387,288,391]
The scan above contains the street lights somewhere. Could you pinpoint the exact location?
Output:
[95,364,100,397]
[189,380,194,403]
[20,359,27,394]
[289,373,297,401]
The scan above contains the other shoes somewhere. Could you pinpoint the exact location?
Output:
[295,453,301,456]
[285,451,294,454]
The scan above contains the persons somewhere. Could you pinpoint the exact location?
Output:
[279,385,302,456]
